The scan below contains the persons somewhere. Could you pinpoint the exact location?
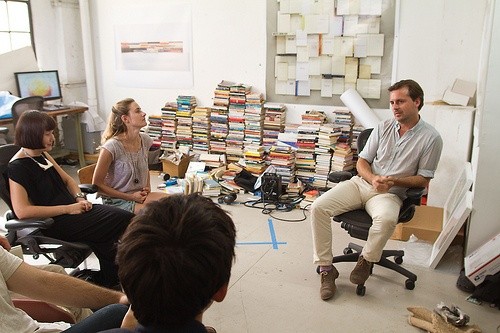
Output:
[310,79,443,299]
[0,245,131,333]
[92,98,172,215]
[116,193,236,333]
[7,110,135,289]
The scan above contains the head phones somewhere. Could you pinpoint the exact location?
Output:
[218,192,237,203]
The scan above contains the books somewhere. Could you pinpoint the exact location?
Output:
[147,80,364,199]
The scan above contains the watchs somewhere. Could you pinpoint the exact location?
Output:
[74,193,84,199]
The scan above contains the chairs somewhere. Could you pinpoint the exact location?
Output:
[317,128,427,297]
[0,145,93,269]
[77,164,96,183]
[0,96,43,145]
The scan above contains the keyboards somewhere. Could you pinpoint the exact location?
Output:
[43,105,71,111]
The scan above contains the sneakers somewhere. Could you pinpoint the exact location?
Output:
[319,264,339,300]
[349,255,372,285]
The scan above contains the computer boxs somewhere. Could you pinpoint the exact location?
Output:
[61,117,101,155]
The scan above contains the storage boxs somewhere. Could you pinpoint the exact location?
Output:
[464,233,500,286]
[441,77,478,106]
[401,204,444,243]
[158,153,196,177]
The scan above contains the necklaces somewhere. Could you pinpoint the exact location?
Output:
[21,148,53,171]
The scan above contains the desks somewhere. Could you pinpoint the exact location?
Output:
[0,103,88,168]
[419,102,475,206]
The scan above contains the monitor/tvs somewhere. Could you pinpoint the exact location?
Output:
[13,69,62,107]
